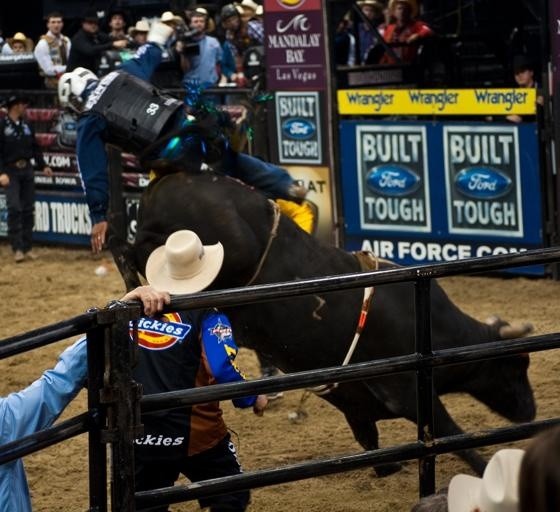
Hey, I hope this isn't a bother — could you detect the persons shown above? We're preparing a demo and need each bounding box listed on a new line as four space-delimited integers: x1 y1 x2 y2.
1 1 266 107
0 285 171 512
58 44 307 267
128 230 268 512
410 427 559 512
331 0 548 130
0 94 53 263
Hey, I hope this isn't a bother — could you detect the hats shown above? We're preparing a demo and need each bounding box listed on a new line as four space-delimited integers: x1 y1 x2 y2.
127 0 264 37
6 32 34 53
355 0 419 19
145 229 225 296
446 448 526 511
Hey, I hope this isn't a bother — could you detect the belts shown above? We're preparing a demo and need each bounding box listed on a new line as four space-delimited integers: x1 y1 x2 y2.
5 158 30 171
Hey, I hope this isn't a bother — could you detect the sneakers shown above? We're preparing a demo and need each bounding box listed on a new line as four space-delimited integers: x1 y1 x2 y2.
264 391 286 410
13 250 40 262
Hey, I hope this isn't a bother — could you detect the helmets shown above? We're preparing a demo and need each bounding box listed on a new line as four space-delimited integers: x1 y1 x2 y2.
58 67 101 122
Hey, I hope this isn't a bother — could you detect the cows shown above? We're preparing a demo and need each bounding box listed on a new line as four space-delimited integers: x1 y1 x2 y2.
107 123 536 479
